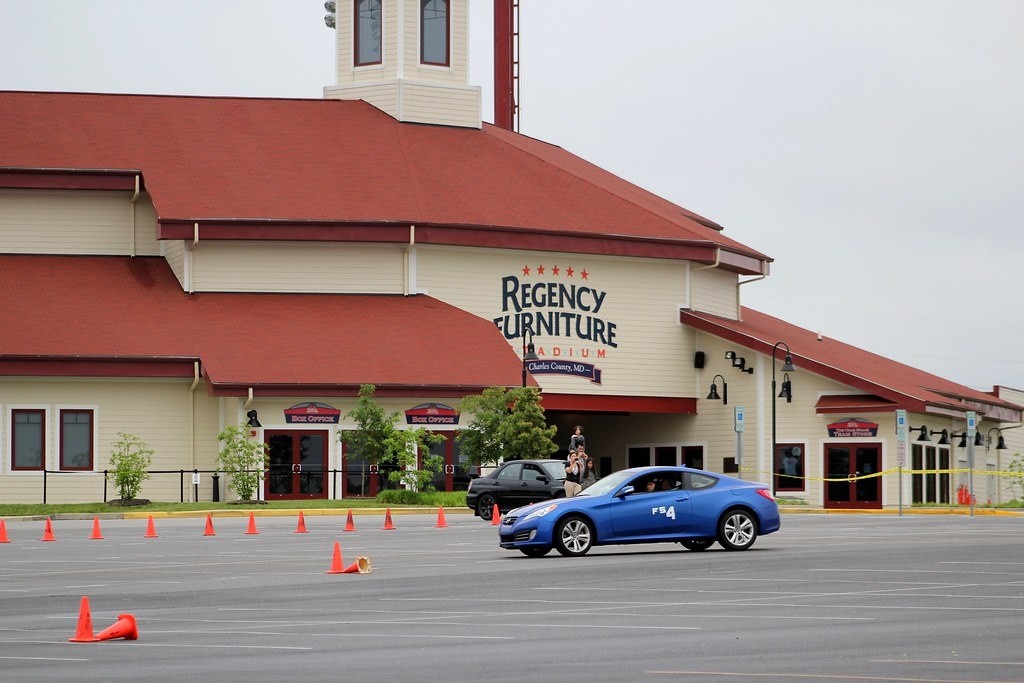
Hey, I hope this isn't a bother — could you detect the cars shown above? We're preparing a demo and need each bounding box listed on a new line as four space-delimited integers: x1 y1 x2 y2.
498 464 782 559
465 458 598 521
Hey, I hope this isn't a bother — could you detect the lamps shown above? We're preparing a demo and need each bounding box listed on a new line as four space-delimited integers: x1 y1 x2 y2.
706 375 727 405
950 432 967 447
974 431 983 446
246 410 262 428
723 351 739 368
732 358 753 375
930 429 950 444
909 425 931 442
986 428 1008 451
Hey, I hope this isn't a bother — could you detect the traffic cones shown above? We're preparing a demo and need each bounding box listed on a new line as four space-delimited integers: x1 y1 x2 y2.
326 542 345 574
244 512 261 534
489 504 500 526
434 506 449 528
40 516 57 541
380 508 396 531
293 511 310 534
344 555 373 574
143 515 159 538
88 517 106 540
0 518 11 543
94 613 139 643
68 595 96 642
201 514 216 536
342 509 358 532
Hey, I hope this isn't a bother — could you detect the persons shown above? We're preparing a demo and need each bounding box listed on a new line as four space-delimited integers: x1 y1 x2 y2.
646 477 659 492
564 425 597 497
662 477 675 490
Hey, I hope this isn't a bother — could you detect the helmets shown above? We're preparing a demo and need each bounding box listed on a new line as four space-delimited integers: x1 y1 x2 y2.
645 476 664 492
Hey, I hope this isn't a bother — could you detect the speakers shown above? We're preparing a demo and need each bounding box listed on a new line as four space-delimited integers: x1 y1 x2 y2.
695 351 704 368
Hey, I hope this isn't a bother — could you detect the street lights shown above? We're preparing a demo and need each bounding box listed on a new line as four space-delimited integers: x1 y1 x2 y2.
771 341 797 497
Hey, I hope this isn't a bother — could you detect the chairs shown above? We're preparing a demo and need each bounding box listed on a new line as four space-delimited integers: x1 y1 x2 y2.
662 479 673 490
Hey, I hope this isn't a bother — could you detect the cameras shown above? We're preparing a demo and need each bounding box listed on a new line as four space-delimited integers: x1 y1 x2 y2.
575 458 578 461
